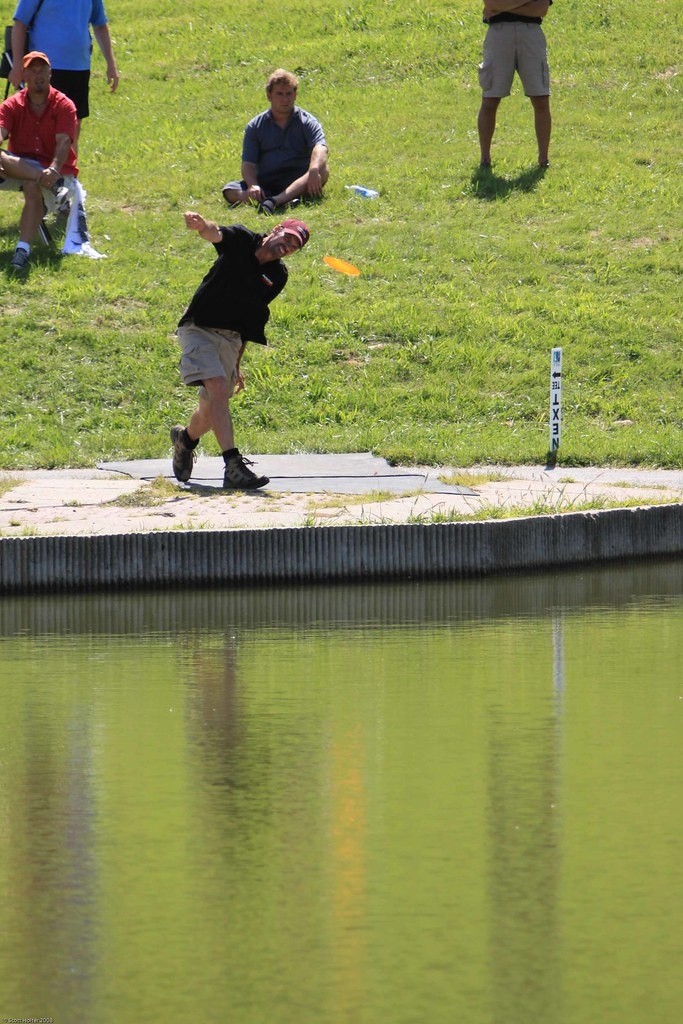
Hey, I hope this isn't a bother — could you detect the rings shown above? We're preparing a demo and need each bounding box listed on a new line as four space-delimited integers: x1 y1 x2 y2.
48 182 50 184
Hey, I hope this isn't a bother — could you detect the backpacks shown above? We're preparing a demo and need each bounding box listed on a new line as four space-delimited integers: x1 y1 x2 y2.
1 26 30 100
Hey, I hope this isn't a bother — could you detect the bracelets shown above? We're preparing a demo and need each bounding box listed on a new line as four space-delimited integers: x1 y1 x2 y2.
48 166 60 176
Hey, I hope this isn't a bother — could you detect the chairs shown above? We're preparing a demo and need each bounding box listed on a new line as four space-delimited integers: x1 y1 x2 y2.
0 25 25 102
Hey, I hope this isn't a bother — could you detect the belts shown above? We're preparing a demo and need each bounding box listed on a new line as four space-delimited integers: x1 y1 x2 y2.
489 12 542 24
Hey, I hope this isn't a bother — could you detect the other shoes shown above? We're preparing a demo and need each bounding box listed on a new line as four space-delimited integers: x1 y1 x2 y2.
286 198 300 208
258 197 275 217
10 248 28 269
50 178 69 207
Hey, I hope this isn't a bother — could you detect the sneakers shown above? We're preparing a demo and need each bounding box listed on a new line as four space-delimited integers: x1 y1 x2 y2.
170 425 197 482
223 455 269 489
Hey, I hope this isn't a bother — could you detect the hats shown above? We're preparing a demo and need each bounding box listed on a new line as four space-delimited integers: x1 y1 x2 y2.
281 219 310 251
22 51 50 68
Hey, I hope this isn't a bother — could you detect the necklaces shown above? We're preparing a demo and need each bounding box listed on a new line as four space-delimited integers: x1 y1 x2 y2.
30 101 46 107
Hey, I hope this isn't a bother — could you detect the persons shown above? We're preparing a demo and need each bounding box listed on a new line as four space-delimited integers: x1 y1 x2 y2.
170 210 310 489
7 0 120 164
0 50 80 270
222 68 329 216
477 0 553 169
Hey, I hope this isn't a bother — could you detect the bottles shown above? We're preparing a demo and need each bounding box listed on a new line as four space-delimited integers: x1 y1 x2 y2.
344 185 379 200
